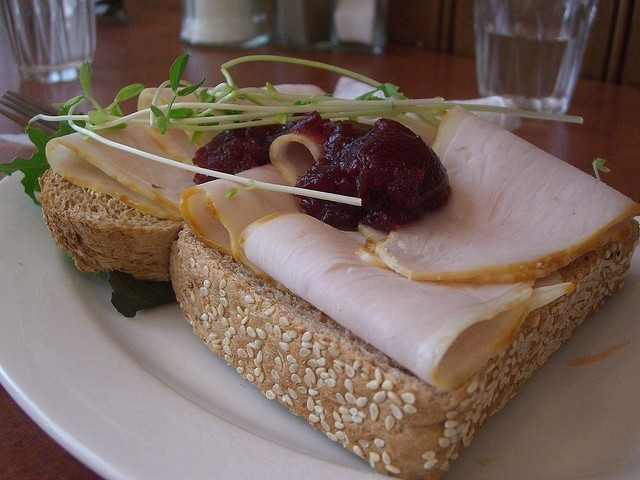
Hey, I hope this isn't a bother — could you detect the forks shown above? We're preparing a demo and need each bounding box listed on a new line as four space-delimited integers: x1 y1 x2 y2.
0 89 88 135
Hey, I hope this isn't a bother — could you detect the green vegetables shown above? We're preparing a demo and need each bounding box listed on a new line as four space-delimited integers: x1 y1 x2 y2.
1 50 584 209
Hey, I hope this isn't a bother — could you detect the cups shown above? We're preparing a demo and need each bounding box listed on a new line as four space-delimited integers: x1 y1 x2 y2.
474 2 597 114
2 1 97 83
